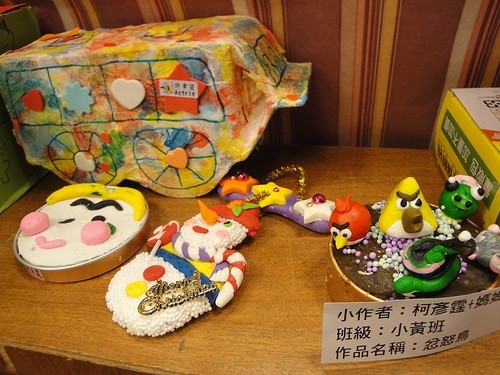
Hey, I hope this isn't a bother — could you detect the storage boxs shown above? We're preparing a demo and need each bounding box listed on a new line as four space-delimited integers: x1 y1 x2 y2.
435 87 500 231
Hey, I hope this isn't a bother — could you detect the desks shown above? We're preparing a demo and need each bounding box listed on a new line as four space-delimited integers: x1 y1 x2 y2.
0 145 500 375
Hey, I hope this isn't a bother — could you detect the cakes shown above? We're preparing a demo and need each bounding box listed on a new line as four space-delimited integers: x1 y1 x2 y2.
329 175 500 304
17 182 148 268
